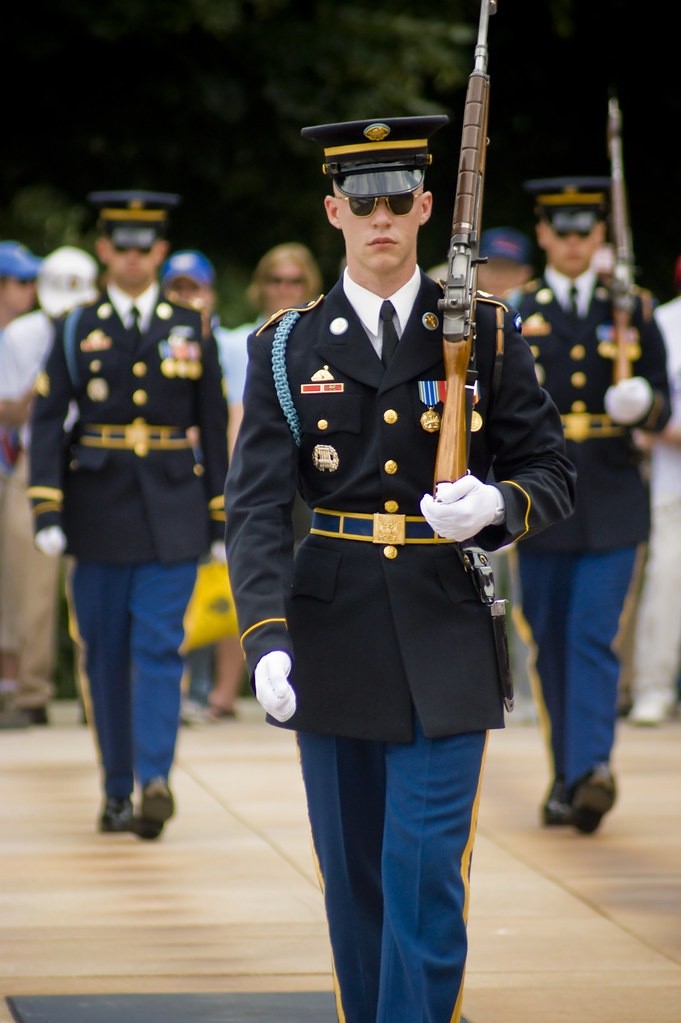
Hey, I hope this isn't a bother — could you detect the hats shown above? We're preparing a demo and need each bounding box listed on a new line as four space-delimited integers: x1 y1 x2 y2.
0 240 43 279
301 115 450 198
522 177 609 217
91 190 178 244
37 247 98 316
478 226 530 261
160 250 213 287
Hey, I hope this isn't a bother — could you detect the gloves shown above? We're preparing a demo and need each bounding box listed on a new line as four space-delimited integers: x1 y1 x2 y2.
254 650 297 723
604 376 652 424
34 524 67 557
420 474 507 542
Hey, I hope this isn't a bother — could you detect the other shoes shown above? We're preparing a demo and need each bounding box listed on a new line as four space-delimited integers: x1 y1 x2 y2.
102 798 136 831
136 780 173 838
544 777 573 822
574 772 614 833
628 689 676 725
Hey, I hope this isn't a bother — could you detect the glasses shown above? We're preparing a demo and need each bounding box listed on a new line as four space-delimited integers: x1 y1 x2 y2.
541 212 601 236
105 237 157 253
337 193 421 218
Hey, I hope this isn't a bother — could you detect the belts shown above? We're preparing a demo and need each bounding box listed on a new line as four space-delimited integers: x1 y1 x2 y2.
78 423 189 449
560 413 623 441
310 508 455 545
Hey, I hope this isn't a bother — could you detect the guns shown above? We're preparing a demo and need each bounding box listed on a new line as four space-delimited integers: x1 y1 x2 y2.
432 0 497 499
607 97 634 382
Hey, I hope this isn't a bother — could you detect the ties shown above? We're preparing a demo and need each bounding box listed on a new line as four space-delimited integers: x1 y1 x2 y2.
379 300 400 369
568 286 577 322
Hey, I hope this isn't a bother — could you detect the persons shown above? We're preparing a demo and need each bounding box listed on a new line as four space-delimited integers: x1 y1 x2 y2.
0 243 40 700
0 238 99 725
478 227 532 297
223 113 576 1023
28 190 228 839
206 241 322 718
501 176 672 835
163 251 217 320
628 294 681 726
